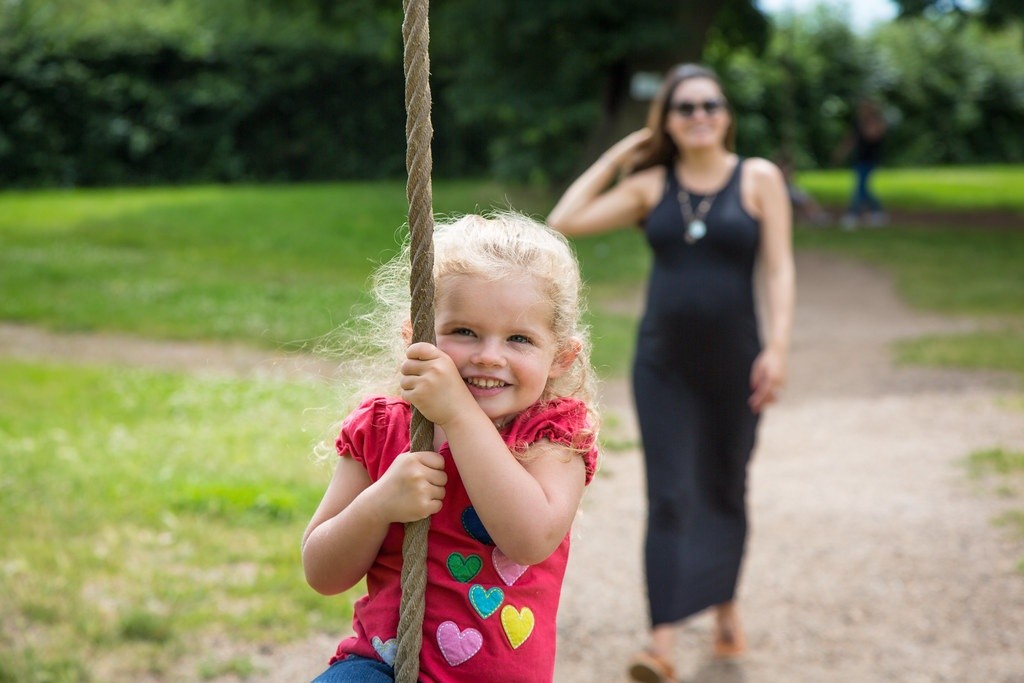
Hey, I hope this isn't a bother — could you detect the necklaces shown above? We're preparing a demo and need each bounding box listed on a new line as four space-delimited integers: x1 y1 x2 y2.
675 164 720 242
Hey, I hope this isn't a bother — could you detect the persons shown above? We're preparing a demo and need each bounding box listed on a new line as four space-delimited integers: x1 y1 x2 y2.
547 65 798 683
778 89 892 234
302 210 604 683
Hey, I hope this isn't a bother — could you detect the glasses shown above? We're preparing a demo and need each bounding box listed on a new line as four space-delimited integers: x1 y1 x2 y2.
670 100 725 117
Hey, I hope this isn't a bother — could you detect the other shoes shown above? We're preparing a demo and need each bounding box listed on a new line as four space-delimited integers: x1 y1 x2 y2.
711 619 745 658
629 655 676 683
